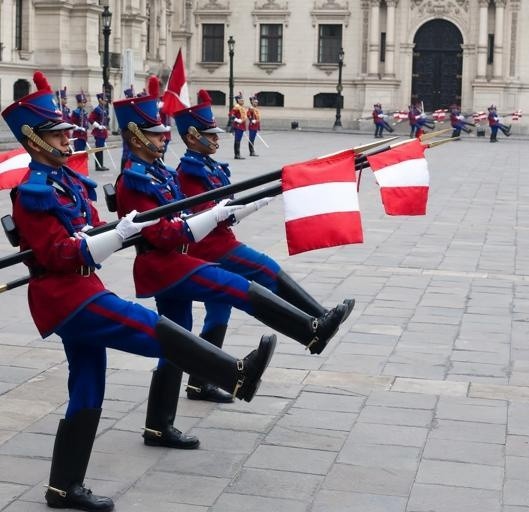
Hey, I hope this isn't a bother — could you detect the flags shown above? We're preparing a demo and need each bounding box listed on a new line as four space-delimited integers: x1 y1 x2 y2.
367 141 435 216
281 154 361 256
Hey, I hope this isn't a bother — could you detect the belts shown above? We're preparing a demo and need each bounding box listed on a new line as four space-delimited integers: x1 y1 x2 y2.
28 265 94 275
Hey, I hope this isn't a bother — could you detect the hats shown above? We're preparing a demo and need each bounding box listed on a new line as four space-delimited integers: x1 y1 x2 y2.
113 76 171 133
124 88 147 98
234 95 259 102
173 89 226 133
56 86 69 99
96 92 109 103
76 94 87 102
1 72 75 141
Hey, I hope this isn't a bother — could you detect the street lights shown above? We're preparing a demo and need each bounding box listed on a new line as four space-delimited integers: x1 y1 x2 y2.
334 46 348 135
225 35 241 132
99 6 118 138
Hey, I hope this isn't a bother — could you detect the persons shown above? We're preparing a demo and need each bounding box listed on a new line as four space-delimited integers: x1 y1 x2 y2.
89 93 110 171
174 102 355 402
122 86 171 164
371 102 512 141
247 97 261 156
71 93 89 149
114 96 349 450
55 91 71 140
1 89 277 512
230 95 246 159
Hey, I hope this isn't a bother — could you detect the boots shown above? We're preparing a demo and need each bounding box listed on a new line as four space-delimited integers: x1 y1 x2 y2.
95 147 109 171
384 124 393 132
234 141 243 159
465 122 475 126
375 128 383 138
155 314 276 402
462 126 471 132
425 124 434 129
410 130 414 138
501 125 511 136
45 408 114 511
451 130 460 141
491 133 497 142
274 270 355 336
249 140 258 156
187 323 233 402
143 370 200 449
247 282 348 354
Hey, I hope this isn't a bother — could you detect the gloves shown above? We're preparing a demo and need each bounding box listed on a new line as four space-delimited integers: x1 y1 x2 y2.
234 118 242 124
69 224 94 242
170 214 192 224
93 121 105 130
86 210 160 264
252 120 256 124
186 199 247 243
72 124 85 132
136 241 189 255
233 197 276 223
